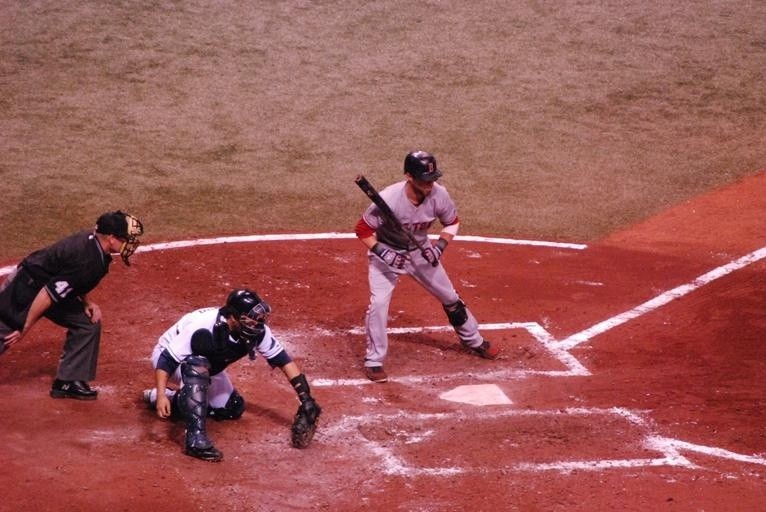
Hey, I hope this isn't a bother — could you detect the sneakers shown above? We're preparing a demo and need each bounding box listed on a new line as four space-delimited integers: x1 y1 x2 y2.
472 339 498 359
143 388 152 403
366 366 388 383
186 446 222 461
50 378 97 401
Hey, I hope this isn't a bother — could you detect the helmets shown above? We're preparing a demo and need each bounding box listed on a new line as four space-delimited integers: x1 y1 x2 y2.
226 288 271 350
404 150 443 182
95 210 144 267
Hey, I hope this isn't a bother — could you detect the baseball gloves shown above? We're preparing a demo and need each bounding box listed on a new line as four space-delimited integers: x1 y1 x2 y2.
292 398 321 449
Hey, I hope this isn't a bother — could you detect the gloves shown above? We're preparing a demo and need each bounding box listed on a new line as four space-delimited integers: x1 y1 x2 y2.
421 237 449 265
370 243 412 271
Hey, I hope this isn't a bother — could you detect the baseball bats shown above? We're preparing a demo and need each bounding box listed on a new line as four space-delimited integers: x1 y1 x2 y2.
354 175 439 267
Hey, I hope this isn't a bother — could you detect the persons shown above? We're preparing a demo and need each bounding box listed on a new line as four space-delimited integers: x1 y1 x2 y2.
0 207 145 401
143 286 323 462
352 149 501 384
